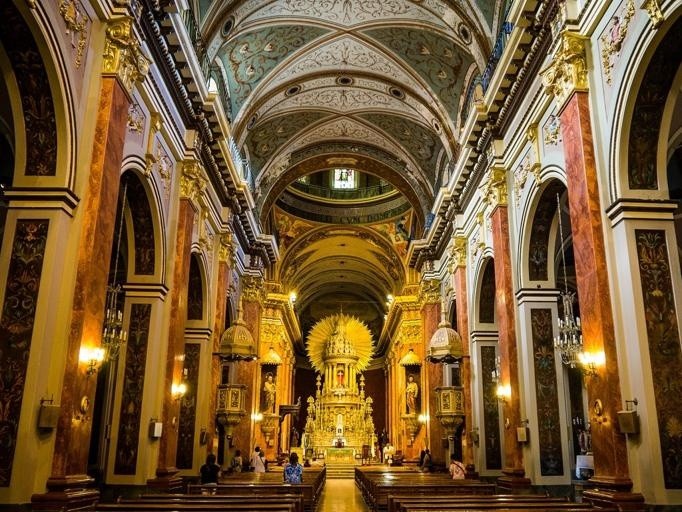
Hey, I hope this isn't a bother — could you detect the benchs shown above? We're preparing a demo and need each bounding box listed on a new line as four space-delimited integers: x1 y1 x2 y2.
91 466 326 512
355 467 601 512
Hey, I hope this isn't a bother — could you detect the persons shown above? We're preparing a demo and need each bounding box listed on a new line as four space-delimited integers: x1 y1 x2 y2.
406 375 418 414
275 447 290 466
302 458 318 467
376 431 404 466
417 450 425 467
449 453 467 480
247 446 270 473
335 439 343 448
283 453 303 484
199 454 220 496
231 450 242 473
291 426 305 447
421 449 432 473
263 376 276 414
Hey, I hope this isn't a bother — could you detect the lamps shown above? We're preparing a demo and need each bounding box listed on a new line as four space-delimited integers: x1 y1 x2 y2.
497 386 508 406
101 175 129 363
86 348 105 379
579 352 598 378
554 193 583 368
175 383 186 401
